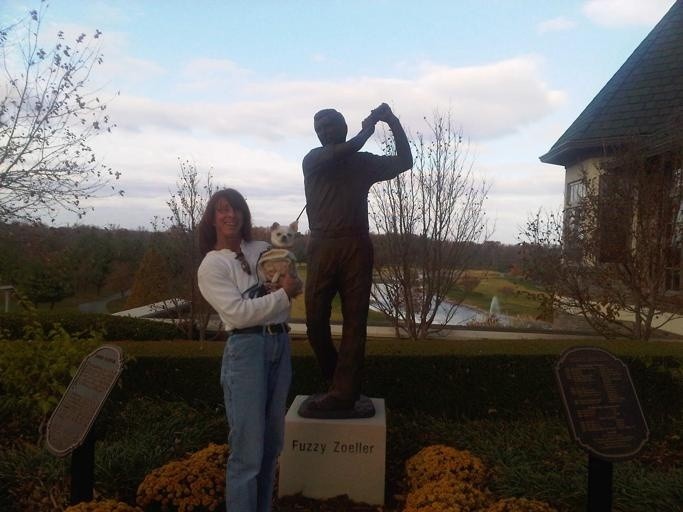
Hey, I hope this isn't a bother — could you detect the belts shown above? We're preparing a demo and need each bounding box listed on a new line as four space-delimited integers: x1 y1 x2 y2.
234 322 290 335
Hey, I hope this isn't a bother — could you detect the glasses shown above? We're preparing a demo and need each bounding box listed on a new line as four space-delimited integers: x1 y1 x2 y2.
234 251 251 275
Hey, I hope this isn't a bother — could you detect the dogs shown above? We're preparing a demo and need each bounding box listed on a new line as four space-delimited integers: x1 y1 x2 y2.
253 220 298 299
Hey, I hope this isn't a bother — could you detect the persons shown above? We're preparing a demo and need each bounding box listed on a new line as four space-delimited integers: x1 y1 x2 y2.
196 188 304 512
301 103 414 411
